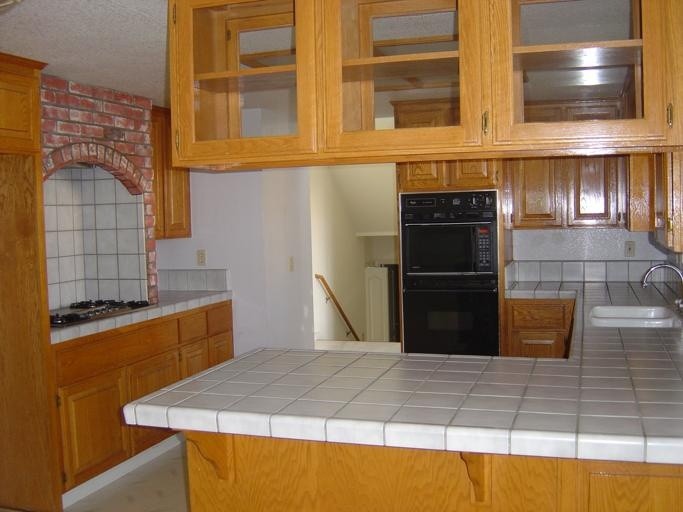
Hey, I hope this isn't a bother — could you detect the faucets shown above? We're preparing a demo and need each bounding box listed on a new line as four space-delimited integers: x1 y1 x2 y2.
640 262 683 312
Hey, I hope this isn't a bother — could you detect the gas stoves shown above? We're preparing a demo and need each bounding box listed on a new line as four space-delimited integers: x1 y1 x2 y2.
49 300 149 328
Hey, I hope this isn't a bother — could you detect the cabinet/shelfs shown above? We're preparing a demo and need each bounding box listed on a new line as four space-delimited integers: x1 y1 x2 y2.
4 53 62 507
621 65 682 252
52 300 233 493
152 105 192 240
490 1 672 159
391 96 507 228
506 298 575 359
509 98 623 228
167 2 481 172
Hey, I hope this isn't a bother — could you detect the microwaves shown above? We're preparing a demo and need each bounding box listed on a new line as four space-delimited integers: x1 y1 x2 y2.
398 191 497 273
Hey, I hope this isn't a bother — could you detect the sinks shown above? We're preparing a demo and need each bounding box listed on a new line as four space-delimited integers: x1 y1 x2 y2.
588 304 675 328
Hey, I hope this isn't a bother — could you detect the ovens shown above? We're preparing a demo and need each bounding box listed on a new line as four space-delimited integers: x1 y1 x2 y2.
399 275 500 357
